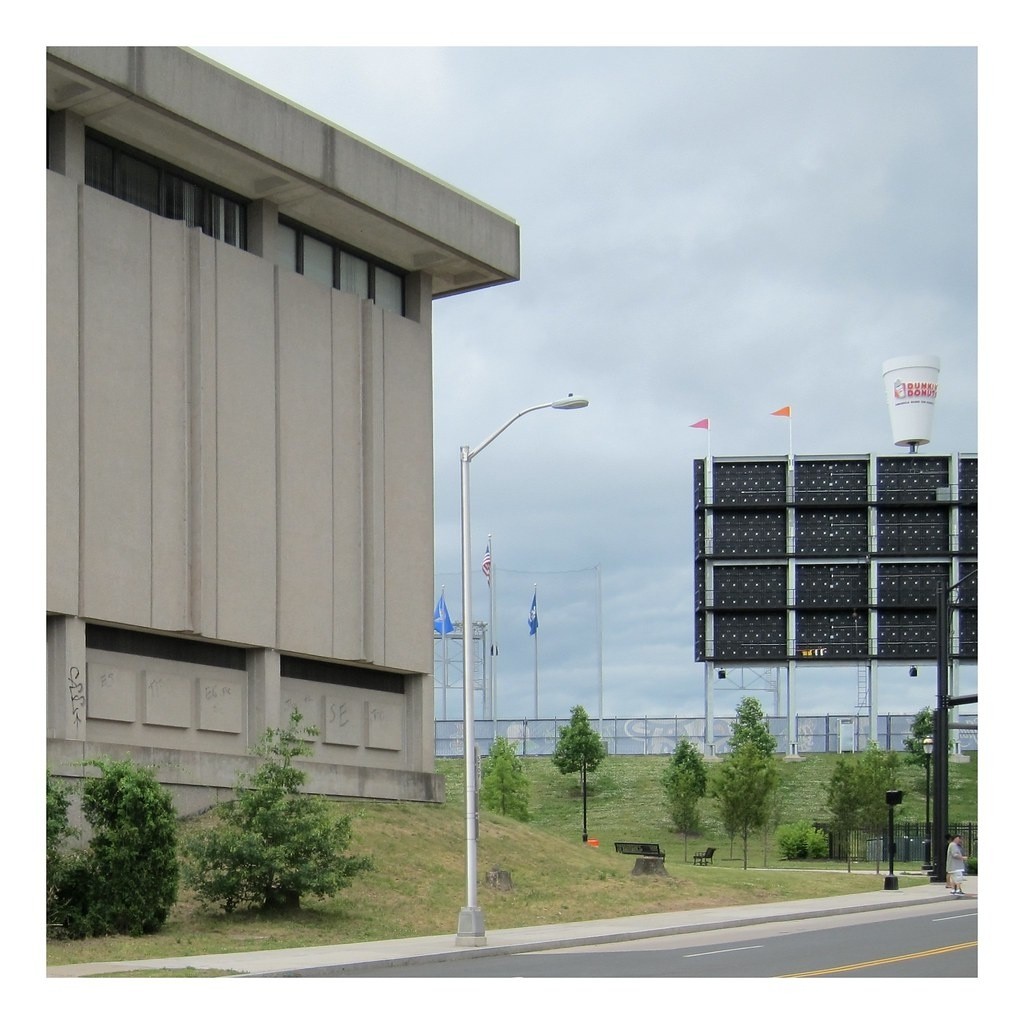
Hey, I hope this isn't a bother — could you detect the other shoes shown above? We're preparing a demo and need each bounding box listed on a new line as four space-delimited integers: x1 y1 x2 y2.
950 889 956 894
955 888 965 895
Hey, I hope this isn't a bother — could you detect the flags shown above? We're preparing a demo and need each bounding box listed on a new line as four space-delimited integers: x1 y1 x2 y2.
482 543 492 586
434 595 453 635
527 592 538 635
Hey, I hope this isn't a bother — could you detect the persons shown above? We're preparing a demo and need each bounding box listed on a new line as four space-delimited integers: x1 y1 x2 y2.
945 833 956 888
946 835 968 896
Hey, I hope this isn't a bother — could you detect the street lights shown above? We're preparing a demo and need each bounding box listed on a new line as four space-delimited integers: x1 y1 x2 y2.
455 395 592 948
922 736 933 871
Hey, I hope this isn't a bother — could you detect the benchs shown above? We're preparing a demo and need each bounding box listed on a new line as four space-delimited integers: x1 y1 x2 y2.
693 847 716 866
614 842 665 862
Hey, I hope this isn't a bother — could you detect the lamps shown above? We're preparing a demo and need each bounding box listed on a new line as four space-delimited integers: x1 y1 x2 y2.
718 667 727 679
909 665 918 677
